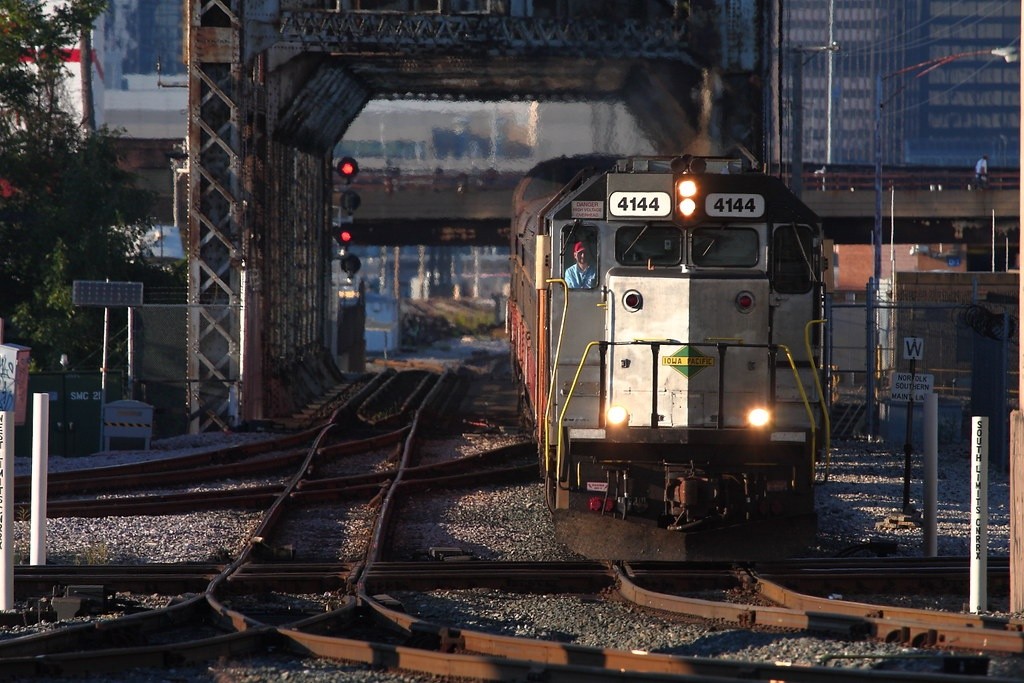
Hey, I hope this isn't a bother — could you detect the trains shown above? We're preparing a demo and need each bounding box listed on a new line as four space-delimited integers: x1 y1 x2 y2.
508 152 836 529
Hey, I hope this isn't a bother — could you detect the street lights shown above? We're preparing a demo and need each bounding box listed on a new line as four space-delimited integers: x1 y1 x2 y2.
873 48 1018 291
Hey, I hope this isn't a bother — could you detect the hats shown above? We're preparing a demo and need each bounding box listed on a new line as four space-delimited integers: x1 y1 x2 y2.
574 241 588 253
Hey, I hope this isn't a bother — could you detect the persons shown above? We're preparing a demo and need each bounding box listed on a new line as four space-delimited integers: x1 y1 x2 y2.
975 155 988 190
565 242 597 289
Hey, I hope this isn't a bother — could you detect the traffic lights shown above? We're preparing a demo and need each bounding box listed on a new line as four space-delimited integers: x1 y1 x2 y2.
341 253 362 275
341 190 360 214
338 157 358 181
336 221 359 247
